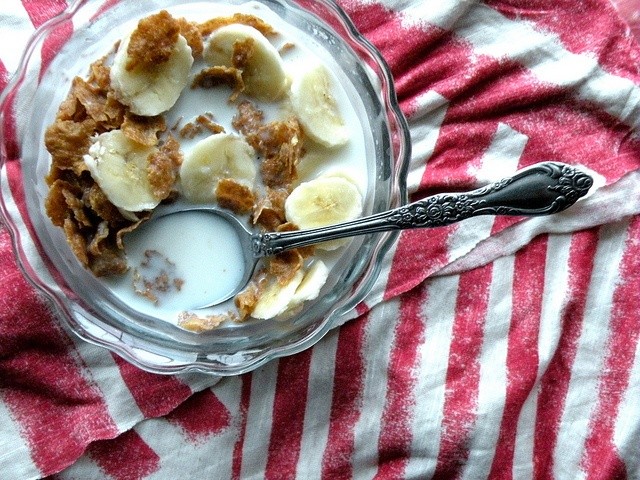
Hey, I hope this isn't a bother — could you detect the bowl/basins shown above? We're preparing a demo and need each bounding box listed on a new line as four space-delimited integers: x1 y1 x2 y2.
0 0 413 379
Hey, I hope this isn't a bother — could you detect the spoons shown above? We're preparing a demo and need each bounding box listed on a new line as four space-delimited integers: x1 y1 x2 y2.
151 163 599 309
